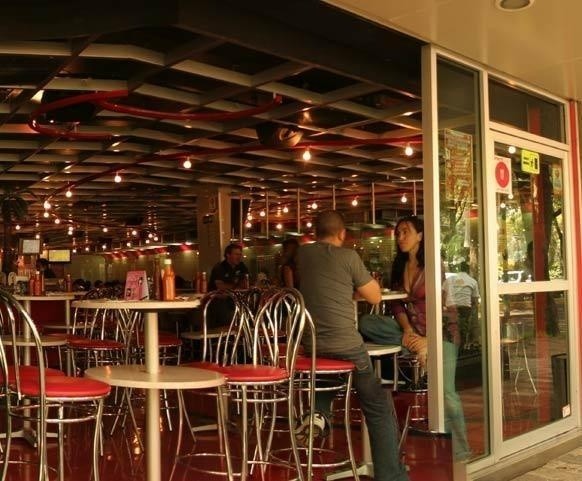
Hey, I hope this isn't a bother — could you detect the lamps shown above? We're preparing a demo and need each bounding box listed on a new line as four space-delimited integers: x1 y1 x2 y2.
302 144 312 160
245 187 408 229
404 142 413 157
41 154 191 218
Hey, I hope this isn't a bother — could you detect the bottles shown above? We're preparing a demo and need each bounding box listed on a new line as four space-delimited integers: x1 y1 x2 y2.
28 270 42 296
195 271 208 294
65 274 73 292
242 272 249 288
152 257 177 300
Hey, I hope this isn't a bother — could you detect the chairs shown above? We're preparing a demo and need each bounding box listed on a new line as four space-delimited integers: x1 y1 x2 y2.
500 316 544 393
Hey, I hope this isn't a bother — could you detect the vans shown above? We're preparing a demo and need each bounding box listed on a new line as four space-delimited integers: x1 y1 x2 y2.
498 267 534 300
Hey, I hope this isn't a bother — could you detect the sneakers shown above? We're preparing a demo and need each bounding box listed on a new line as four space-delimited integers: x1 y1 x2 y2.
294 413 325 448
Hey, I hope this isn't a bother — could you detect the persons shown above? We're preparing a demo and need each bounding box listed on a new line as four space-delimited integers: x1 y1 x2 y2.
278 239 299 319
442 260 478 351
93 279 120 290
208 244 251 289
356 215 474 467
287 209 414 481
35 259 56 279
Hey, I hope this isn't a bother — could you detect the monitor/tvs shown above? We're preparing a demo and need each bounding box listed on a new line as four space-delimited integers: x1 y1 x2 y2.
18 238 43 255
48 249 72 264
